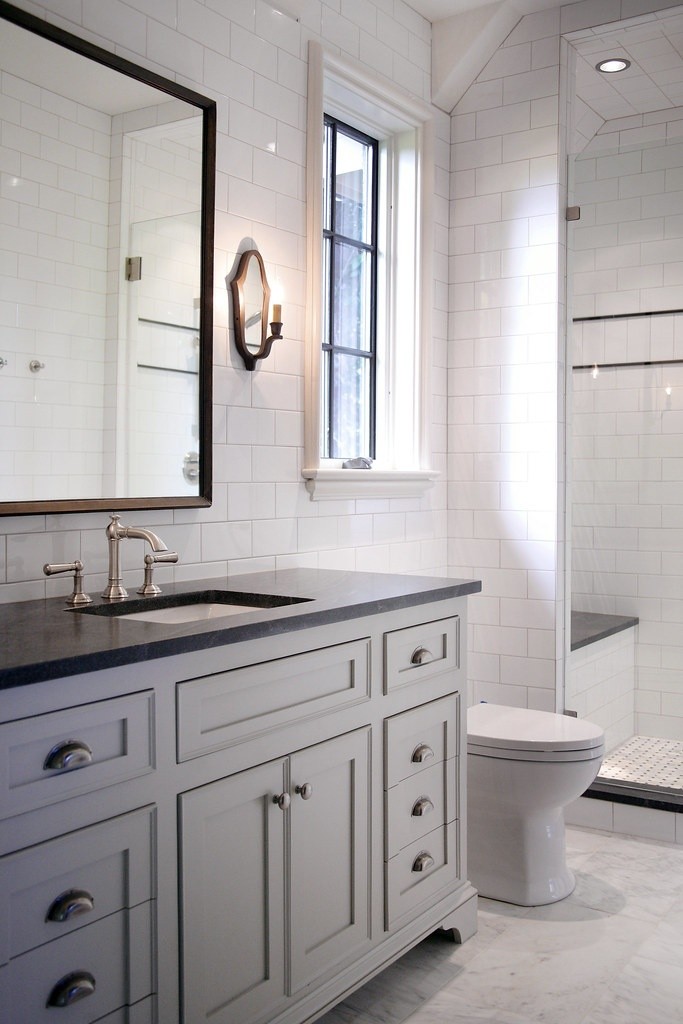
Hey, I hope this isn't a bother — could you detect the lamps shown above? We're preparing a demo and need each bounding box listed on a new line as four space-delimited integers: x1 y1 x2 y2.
269 282 284 335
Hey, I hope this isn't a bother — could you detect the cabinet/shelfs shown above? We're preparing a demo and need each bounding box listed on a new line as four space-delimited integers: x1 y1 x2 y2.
0 594 480 1024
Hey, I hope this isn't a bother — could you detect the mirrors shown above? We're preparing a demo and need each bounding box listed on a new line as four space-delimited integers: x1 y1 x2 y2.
0 0 218 517
229 249 272 371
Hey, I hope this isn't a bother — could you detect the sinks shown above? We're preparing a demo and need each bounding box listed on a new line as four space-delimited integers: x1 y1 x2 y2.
63 589 316 625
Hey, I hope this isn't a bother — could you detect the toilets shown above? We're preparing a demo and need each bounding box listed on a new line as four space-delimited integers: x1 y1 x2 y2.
466 700 606 906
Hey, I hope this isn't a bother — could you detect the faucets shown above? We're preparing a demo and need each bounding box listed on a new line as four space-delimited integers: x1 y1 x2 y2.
101 513 167 598
